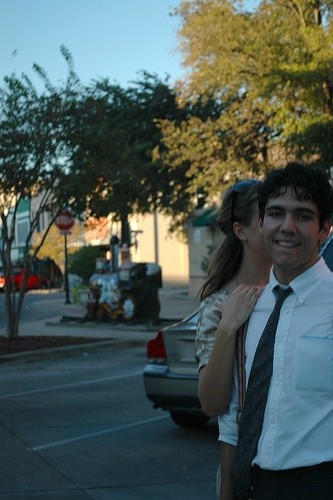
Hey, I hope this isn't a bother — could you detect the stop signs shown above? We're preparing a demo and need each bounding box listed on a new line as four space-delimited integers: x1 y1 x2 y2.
53 210 74 230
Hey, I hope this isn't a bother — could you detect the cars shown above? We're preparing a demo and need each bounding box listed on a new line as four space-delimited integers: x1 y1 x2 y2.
0 266 54 293
142 292 213 430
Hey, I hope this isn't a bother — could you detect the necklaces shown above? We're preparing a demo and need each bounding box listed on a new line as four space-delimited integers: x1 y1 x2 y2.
236 276 240 287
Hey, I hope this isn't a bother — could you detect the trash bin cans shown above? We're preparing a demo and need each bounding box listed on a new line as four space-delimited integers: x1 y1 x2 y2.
117 262 164 325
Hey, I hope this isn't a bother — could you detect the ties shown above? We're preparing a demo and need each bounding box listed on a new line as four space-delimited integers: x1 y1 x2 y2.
230 286 292 480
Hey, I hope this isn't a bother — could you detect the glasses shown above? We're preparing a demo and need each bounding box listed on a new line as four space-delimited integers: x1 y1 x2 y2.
230 180 261 224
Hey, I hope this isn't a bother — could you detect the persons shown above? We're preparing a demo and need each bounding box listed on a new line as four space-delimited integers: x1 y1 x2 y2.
113 263 160 325
216 162 333 500
120 243 131 263
192 178 272 500
